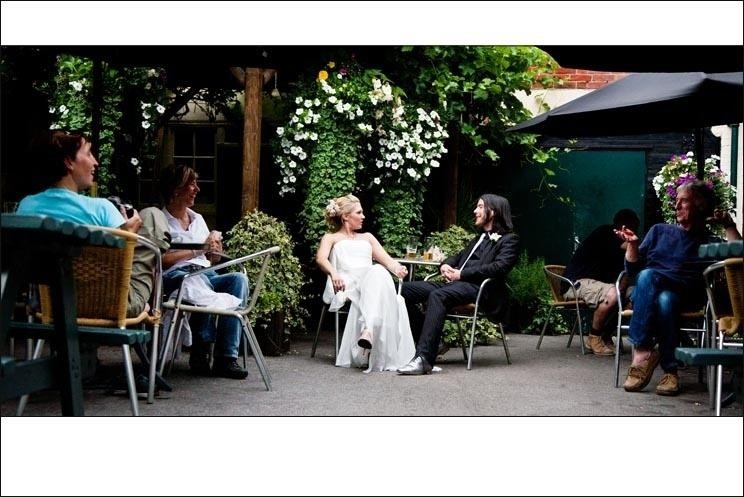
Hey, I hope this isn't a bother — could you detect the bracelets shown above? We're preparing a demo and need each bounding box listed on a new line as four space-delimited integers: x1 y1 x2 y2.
191 250 196 258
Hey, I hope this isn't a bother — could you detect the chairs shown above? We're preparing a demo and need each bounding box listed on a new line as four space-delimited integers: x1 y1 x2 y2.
1 225 743 416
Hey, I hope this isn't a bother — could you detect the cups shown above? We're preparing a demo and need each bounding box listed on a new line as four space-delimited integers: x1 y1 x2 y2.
424 244 433 260
406 244 417 259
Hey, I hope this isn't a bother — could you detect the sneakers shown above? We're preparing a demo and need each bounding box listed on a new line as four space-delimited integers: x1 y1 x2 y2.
212 355 249 381
188 342 212 379
656 373 682 397
622 349 659 392
584 335 619 356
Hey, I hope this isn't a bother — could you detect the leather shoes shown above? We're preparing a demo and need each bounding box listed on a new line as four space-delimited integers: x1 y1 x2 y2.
395 355 432 375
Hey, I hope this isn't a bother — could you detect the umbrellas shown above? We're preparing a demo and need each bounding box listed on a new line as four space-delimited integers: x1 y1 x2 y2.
504 71 743 182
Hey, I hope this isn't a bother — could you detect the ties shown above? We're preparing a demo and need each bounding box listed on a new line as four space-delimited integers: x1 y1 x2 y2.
458 233 486 271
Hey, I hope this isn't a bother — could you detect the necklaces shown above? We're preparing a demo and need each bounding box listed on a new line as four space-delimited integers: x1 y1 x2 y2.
167 205 190 231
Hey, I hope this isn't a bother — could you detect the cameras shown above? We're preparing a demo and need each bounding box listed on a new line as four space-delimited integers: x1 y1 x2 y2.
108 195 134 219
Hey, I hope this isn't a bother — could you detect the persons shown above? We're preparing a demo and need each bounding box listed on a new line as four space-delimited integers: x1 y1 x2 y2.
159 164 249 379
560 208 642 356
314 195 441 375
14 131 160 400
613 181 742 396
394 193 520 376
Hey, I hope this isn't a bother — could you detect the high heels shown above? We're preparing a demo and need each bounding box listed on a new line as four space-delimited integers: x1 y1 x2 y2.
358 329 373 357
